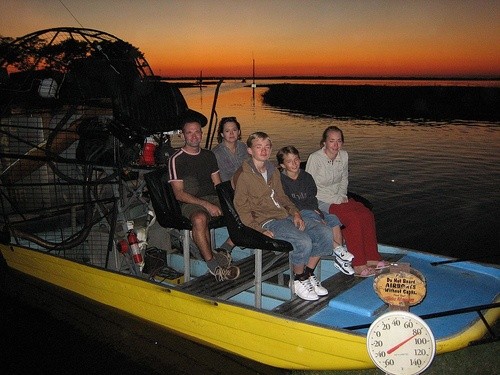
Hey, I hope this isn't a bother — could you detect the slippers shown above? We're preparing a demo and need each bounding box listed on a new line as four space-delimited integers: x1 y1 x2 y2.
353 266 377 277
371 259 391 270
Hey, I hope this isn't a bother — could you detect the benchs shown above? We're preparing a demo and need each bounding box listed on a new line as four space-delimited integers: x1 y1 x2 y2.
143 167 227 283
215 160 374 309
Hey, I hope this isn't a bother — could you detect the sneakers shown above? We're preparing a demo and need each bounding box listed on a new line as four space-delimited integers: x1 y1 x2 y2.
333 256 355 275
305 269 329 296
207 266 240 281
334 246 355 263
288 274 321 301
210 247 233 266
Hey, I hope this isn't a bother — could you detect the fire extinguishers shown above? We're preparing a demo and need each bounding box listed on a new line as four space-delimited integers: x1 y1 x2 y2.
126 229 142 264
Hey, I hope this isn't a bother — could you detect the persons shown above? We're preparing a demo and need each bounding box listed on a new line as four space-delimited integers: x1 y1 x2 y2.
276 145 355 276
212 117 251 182
305 126 391 278
167 117 241 281
231 132 334 301
